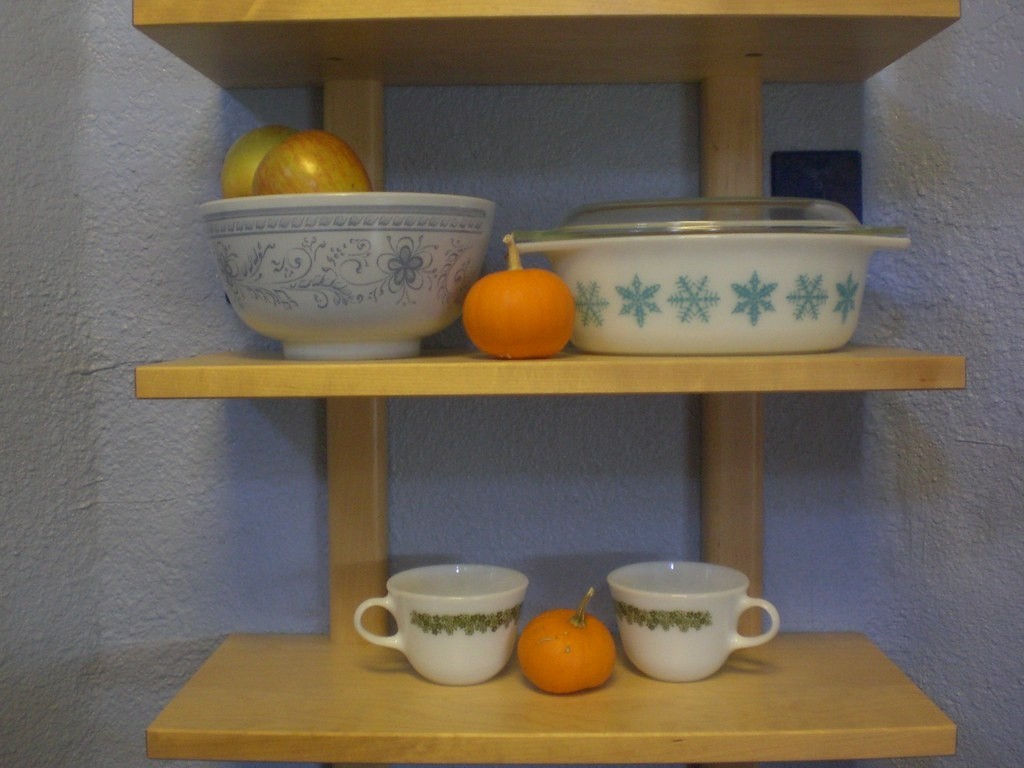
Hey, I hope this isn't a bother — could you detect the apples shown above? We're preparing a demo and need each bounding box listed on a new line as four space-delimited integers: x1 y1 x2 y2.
218 122 372 198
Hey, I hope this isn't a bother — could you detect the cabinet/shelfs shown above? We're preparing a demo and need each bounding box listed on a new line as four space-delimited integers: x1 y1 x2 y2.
132 0 964 768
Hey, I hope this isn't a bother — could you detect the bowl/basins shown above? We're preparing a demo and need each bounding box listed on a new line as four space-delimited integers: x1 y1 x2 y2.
200 192 496 360
514 233 912 355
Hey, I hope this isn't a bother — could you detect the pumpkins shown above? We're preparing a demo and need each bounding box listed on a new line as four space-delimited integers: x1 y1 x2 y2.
461 233 575 360
517 587 616 693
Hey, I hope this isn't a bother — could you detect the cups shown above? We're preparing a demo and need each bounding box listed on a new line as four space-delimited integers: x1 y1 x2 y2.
606 560 781 683
353 563 530 686
